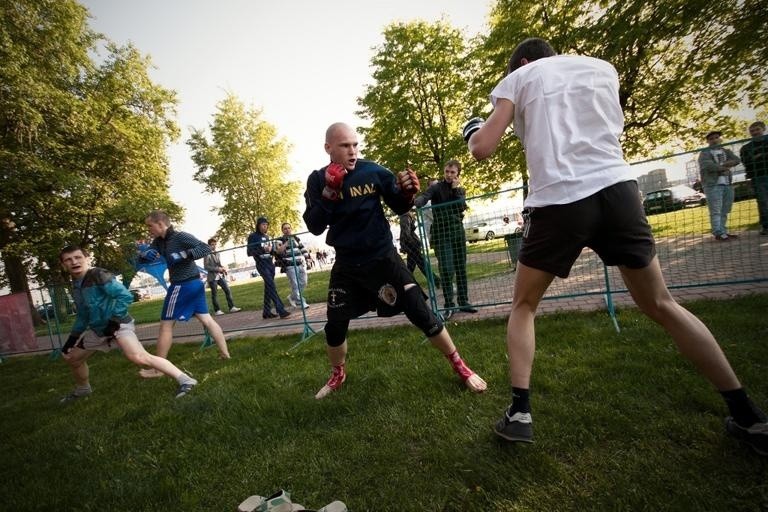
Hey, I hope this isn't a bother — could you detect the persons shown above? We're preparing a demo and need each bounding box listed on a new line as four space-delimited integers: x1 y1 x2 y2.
308 247 336 269
399 210 441 290
35 298 48 311
461 37 768 445
693 178 704 193
246 217 291 319
302 122 488 399
420 177 458 295
139 210 231 379
275 223 311 310
59 245 199 403
414 159 478 322
696 130 741 243
203 239 242 316
738 120 768 235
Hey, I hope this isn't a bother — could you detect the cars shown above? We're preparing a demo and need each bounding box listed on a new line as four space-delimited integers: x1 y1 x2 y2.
643 185 706 215
36 300 78 320
130 289 149 299
731 173 756 202
464 218 524 241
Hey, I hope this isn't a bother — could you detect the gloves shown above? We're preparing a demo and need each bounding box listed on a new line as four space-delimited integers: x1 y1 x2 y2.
397 168 421 205
168 250 188 265
461 116 486 141
141 247 159 264
323 165 346 199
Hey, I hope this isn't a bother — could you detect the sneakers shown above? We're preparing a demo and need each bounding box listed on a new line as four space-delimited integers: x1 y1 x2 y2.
214 310 225 316
287 294 298 308
296 304 309 310
719 411 768 454
487 415 537 444
229 306 243 312
716 235 732 242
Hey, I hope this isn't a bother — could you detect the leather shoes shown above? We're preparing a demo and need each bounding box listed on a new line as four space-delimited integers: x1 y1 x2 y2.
460 303 478 312
443 306 457 320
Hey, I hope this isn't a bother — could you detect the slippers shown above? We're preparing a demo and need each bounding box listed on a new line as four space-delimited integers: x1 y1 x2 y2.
175 381 198 402
